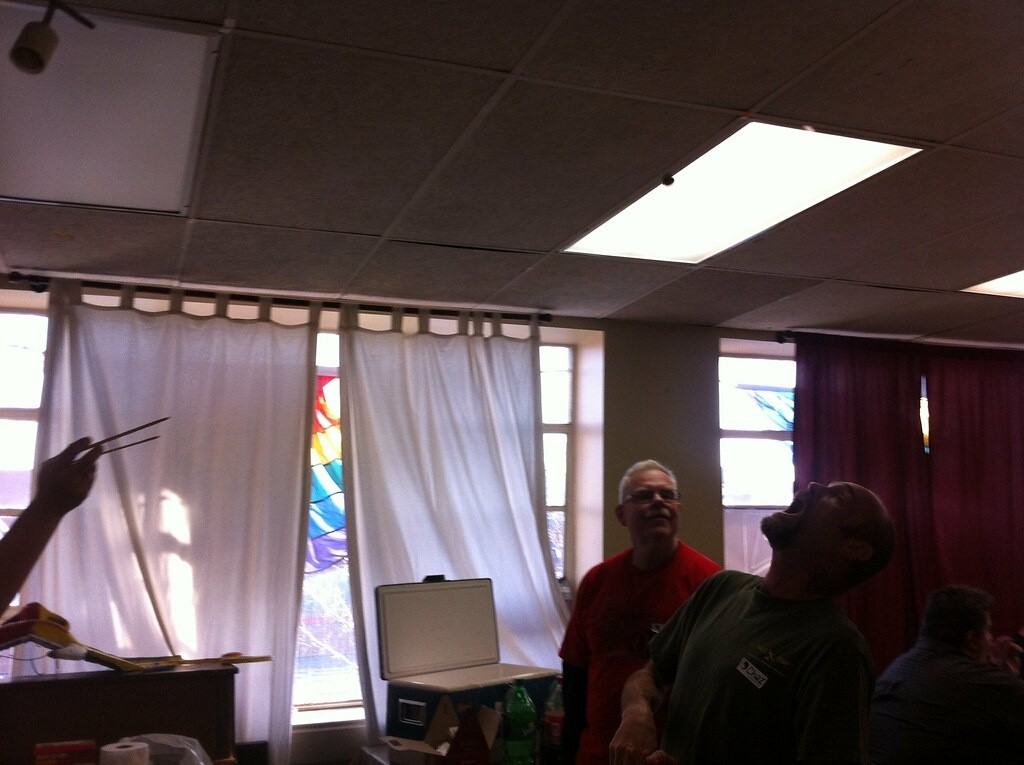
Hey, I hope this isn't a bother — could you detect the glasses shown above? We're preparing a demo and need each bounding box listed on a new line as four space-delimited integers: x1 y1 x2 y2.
622 489 684 504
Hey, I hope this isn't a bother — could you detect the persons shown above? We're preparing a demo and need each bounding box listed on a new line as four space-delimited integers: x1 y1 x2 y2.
606 479 895 765
558 458 724 765
864 581 1024 765
0 436 105 621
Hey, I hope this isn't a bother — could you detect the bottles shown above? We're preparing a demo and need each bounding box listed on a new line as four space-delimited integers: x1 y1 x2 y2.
558 575 577 614
504 679 539 765
544 676 568 765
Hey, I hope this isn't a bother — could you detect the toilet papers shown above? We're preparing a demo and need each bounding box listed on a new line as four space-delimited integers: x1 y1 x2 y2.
99 743 151 765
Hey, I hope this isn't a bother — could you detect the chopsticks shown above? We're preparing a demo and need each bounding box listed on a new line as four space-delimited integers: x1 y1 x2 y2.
74 416 172 464
